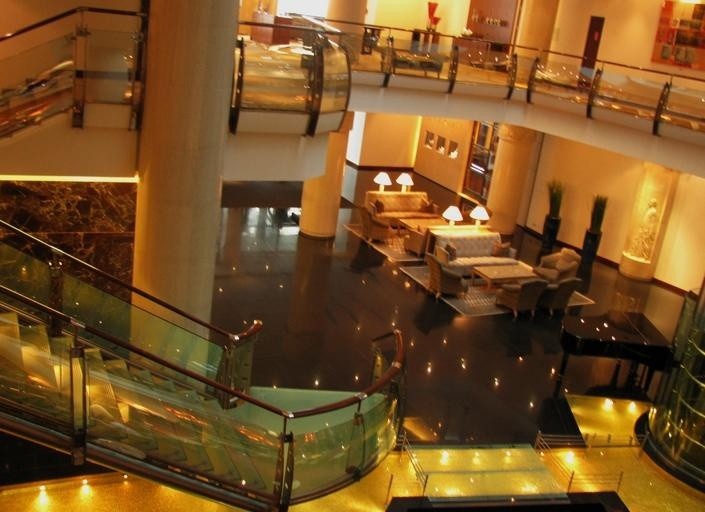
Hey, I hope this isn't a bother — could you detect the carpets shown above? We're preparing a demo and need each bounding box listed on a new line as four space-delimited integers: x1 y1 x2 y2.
220 179 356 210
342 222 426 263
398 261 596 318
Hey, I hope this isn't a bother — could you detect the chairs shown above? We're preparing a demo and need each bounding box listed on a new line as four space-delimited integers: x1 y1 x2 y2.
358 187 585 319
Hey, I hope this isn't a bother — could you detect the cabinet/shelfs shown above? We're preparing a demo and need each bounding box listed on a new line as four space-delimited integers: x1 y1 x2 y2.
452 32 514 70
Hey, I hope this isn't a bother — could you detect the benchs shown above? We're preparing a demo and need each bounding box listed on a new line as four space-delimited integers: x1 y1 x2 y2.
380 46 449 80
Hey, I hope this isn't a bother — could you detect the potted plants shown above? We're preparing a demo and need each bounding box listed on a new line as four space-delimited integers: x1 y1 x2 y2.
583 195 608 264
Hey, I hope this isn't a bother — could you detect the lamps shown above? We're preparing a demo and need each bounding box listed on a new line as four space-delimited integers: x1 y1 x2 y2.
396 172 414 192
373 171 393 191
